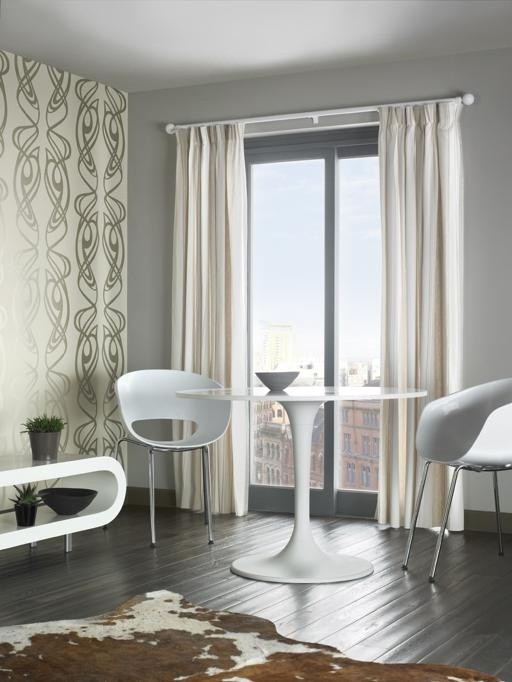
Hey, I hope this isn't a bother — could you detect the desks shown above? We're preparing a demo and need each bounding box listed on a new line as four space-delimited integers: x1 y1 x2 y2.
176 385 428 585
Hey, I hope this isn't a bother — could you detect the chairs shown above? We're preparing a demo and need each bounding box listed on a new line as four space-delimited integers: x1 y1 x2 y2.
400 377 512 583
114 369 232 548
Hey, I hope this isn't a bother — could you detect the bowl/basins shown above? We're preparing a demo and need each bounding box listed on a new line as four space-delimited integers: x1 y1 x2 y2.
38 487 98 516
256 372 299 394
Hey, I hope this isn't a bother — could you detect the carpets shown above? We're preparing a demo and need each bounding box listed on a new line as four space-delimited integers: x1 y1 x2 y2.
0 589 504 682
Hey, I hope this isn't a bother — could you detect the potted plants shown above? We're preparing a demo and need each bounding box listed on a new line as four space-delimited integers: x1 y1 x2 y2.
20 416 64 460
8 482 46 526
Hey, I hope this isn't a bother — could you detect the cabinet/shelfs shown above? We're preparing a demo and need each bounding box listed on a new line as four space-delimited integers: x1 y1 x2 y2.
1 454 127 553
